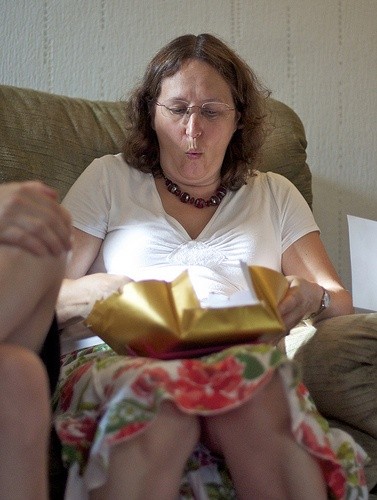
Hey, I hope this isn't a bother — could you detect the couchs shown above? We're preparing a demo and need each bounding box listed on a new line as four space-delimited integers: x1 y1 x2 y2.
0 84 377 500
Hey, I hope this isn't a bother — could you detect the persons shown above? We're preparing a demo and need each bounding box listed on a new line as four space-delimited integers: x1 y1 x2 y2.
0 180 73 499
60 33 372 498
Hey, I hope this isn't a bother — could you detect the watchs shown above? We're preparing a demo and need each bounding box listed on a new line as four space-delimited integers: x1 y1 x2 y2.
310 286 330 317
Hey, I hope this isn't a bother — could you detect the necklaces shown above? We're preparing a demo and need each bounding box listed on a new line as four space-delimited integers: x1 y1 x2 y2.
162 175 226 208
